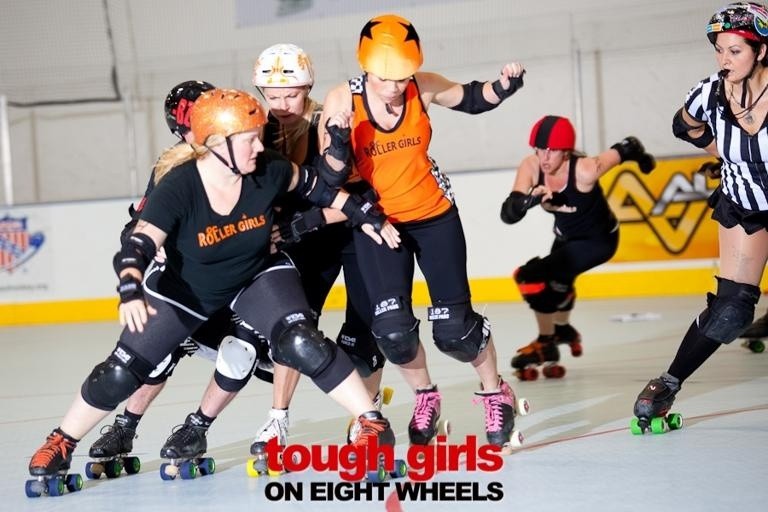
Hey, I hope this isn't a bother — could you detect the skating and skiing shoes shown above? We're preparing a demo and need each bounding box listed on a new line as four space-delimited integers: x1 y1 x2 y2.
510 333 565 380
158 413 217 482
344 411 406 487
245 407 299 480
548 322 583 360
629 369 683 436
86 413 140 482
405 384 451 447
24 427 84 499
347 384 395 438
470 375 530 455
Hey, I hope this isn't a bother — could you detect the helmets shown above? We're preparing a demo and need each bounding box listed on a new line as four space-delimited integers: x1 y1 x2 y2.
530 115 578 152
184 88 268 176
250 41 317 125
162 79 214 141
706 0 766 45
356 13 425 116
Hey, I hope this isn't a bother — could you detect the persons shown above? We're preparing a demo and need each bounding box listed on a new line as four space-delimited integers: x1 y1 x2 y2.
23 86 402 500
314 11 531 455
244 41 385 482
85 79 268 484
630 0 768 434
499 114 656 382
